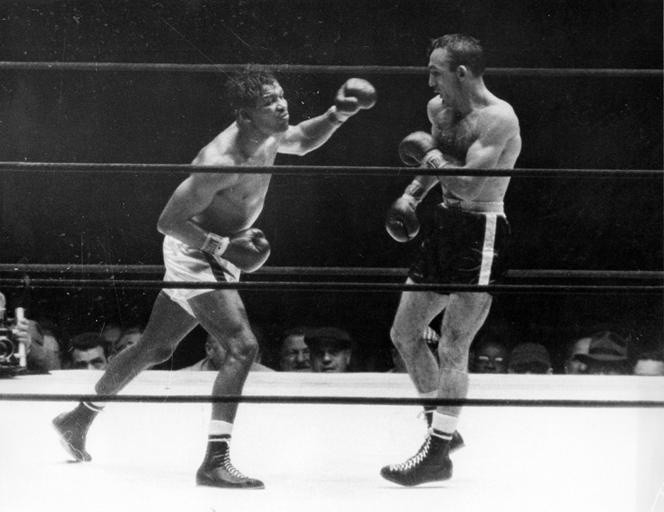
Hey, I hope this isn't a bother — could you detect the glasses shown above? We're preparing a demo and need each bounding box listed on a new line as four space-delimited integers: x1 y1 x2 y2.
511 362 549 374
477 354 505 364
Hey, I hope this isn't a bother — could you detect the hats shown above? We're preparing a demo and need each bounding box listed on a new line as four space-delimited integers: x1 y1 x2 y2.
571 331 627 362
422 325 440 343
304 326 351 350
509 343 550 365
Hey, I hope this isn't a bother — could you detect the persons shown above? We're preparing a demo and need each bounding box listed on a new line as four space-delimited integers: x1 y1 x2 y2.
380 32 526 489
48 62 381 491
0 311 662 377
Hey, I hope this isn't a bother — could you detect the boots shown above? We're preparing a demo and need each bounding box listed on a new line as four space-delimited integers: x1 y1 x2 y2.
195 434 265 490
379 405 466 488
50 401 104 462
